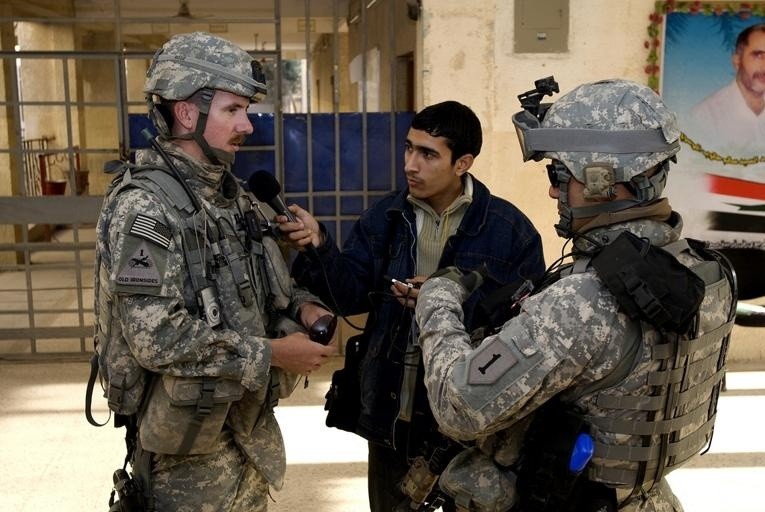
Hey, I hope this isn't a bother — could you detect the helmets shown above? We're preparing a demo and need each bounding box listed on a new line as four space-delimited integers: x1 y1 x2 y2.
143 31 267 102
541 79 680 200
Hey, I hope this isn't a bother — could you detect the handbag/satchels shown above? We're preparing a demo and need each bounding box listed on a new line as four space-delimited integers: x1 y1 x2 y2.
323 333 366 434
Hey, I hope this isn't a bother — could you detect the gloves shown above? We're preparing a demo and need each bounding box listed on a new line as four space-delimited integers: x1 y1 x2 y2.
425 265 484 302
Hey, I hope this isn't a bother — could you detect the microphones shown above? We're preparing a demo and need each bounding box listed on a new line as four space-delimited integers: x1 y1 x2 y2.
245 168 319 258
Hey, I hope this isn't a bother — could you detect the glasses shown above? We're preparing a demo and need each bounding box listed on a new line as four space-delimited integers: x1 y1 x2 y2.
310 314 337 345
545 163 570 186
512 103 666 163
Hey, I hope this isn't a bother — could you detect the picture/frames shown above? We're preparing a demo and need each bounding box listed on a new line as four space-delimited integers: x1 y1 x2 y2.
651 3 765 250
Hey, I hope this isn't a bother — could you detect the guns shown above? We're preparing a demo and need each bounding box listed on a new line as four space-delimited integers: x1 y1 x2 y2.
113 470 142 511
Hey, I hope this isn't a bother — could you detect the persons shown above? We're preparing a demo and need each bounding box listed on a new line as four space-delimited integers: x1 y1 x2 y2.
412 75 741 511
267 100 547 509
89 29 339 511
689 25 765 179
